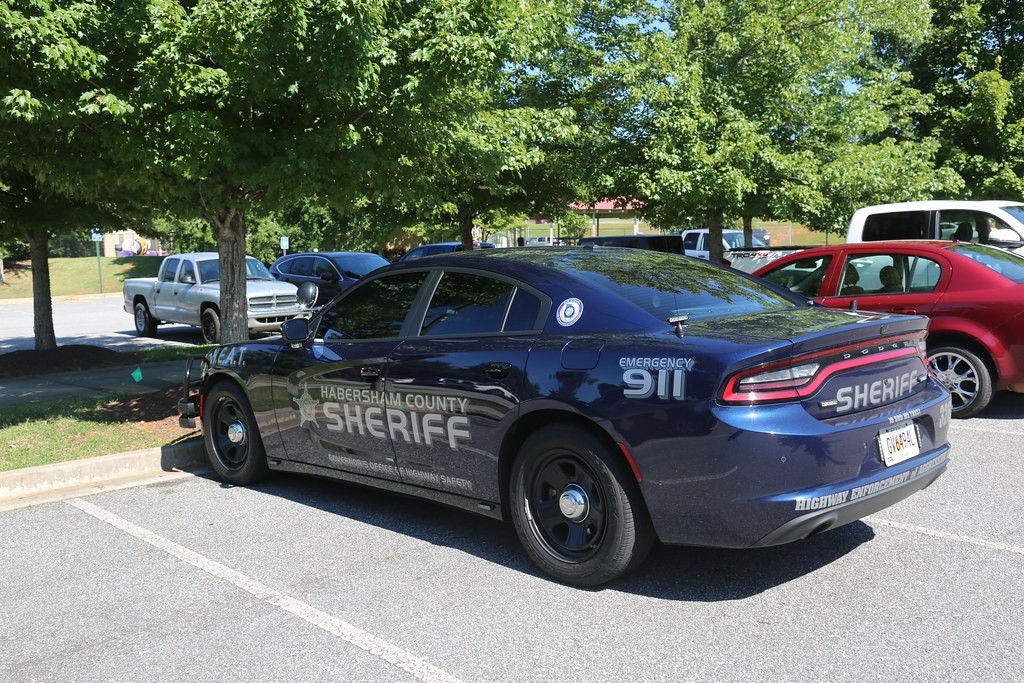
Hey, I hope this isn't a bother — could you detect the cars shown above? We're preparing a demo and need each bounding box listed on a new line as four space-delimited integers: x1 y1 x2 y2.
921 221 979 241
749 239 1024 419
750 227 770 245
267 251 393 309
177 244 952 592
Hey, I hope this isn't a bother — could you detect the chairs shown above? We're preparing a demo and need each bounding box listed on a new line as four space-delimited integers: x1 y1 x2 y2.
954 222 973 242
878 265 903 293
205 270 219 280
840 263 864 296
424 295 474 334
473 292 528 330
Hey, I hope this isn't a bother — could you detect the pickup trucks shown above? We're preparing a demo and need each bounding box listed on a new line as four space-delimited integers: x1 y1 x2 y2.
123 250 314 345
726 200 1024 292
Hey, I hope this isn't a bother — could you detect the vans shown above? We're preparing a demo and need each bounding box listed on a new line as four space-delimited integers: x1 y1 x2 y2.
682 228 770 264
397 242 495 263
525 235 558 247
